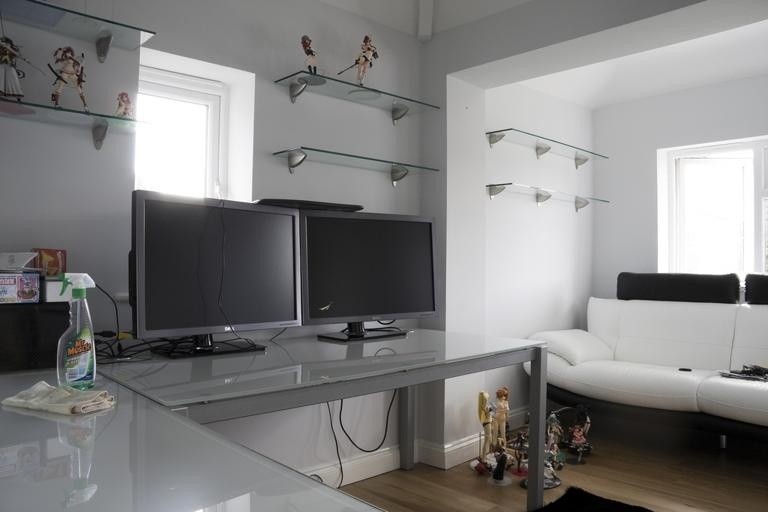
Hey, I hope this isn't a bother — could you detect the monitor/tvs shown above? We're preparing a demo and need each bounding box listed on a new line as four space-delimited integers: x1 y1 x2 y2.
303 210 438 343
128 190 301 359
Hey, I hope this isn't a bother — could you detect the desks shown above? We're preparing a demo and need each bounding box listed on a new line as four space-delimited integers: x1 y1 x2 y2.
3 365 386 512
93 329 547 509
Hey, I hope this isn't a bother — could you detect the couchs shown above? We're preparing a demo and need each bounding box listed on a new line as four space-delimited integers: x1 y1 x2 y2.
522 272 768 446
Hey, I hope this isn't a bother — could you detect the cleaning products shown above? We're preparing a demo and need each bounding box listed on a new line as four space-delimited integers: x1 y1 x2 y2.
56 272 96 390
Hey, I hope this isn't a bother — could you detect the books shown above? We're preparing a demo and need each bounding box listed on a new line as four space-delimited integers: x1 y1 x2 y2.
0 251 42 274
721 372 768 382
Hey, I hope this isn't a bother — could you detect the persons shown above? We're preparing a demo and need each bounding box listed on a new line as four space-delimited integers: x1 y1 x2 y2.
114 92 131 118
0 36 44 103
299 34 317 75
47 44 88 113
470 385 593 489
337 35 380 87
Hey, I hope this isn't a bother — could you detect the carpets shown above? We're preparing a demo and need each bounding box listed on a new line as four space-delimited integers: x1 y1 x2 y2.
544 486 654 511
